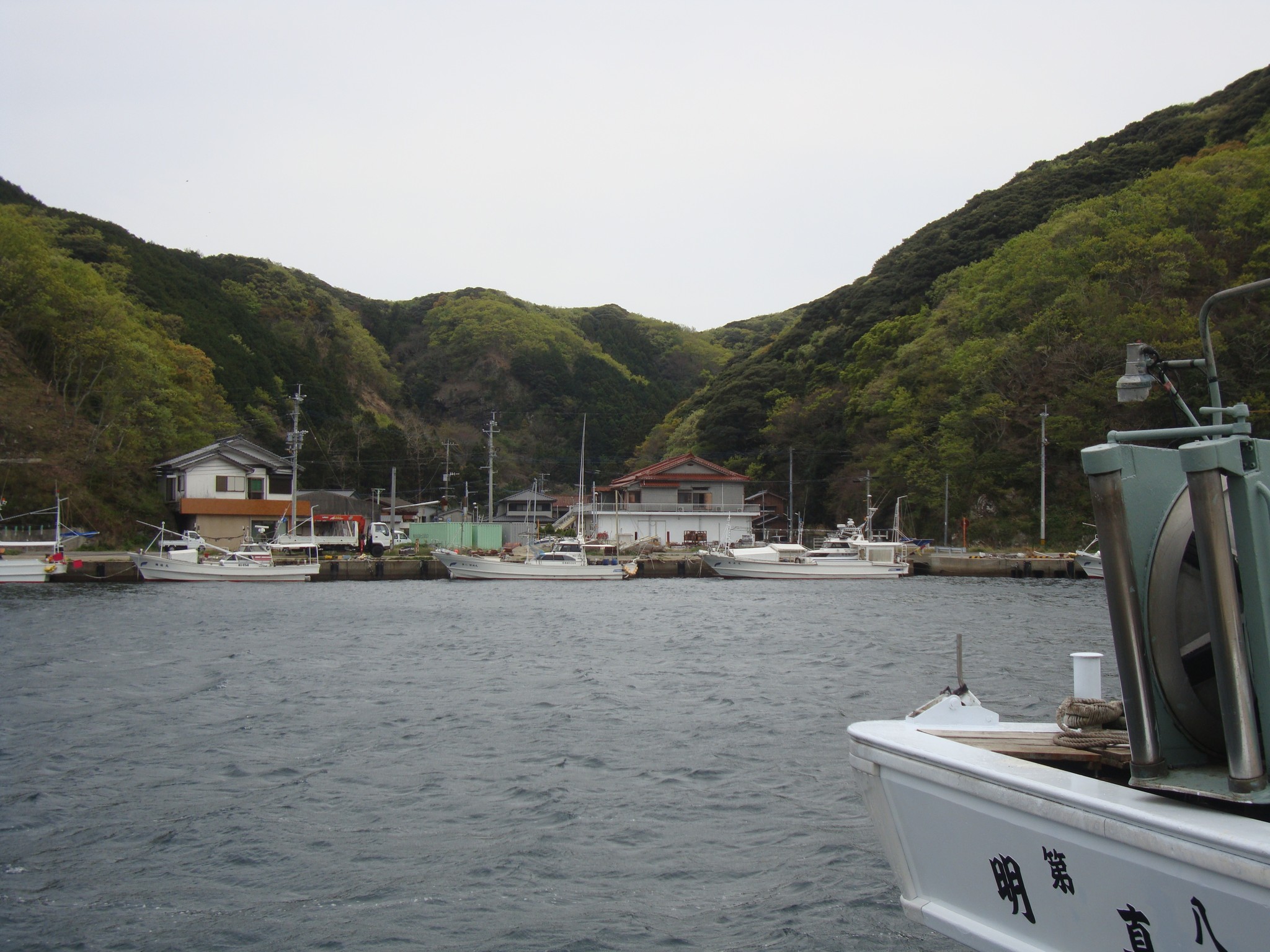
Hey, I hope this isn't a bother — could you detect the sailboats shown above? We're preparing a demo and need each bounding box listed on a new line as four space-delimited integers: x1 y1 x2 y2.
0 457 101 584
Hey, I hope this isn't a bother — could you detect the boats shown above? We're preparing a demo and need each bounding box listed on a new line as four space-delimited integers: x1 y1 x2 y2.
695 494 935 581
430 533 638 581
851 274 1269 952
1067 520 1105 580
127 514 321 584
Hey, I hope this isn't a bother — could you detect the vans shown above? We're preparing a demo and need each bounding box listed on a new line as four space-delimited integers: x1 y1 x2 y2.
376 530 412 547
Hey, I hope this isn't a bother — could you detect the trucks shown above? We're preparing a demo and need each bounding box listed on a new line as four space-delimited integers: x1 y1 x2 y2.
277 513 393 558
